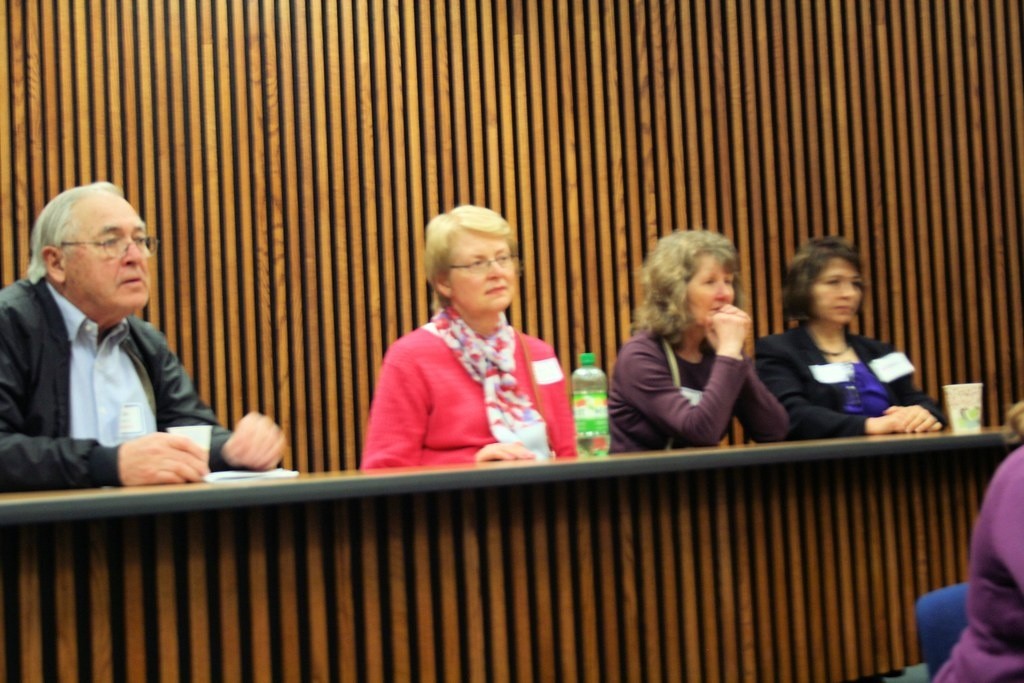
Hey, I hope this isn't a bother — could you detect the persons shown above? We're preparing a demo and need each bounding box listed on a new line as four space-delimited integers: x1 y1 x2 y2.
753 236 947 439
607 230 789 456
0 182 284 491
931 401 1024 683
361 205 578 470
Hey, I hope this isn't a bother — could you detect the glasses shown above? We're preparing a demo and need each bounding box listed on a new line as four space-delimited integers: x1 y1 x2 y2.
447 254 520 276
60 236 157 258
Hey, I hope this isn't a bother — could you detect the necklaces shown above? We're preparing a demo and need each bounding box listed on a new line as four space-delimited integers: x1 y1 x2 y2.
821 347 850 356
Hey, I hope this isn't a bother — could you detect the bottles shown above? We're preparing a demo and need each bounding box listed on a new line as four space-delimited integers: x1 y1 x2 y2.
570 352 611 458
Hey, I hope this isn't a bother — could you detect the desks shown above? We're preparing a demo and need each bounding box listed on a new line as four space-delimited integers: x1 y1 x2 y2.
0 429 1013 683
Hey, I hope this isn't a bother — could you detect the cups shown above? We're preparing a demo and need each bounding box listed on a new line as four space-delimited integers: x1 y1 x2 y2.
942 382 984 436
168 425 212 478
